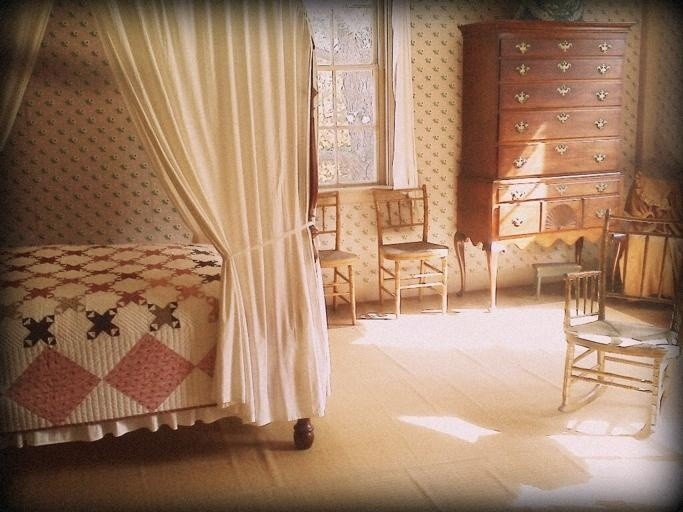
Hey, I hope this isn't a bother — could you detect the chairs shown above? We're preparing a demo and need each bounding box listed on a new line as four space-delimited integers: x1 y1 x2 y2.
558 213 682 432
308 186 450 327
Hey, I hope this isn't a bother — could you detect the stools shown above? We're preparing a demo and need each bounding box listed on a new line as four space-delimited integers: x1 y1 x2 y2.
530 262 582 299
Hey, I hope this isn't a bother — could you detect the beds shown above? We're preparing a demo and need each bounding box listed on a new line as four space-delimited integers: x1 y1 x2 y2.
1 242 324 448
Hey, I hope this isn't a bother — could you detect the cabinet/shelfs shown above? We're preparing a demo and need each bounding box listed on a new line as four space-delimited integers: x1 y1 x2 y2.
452 20 625 315
